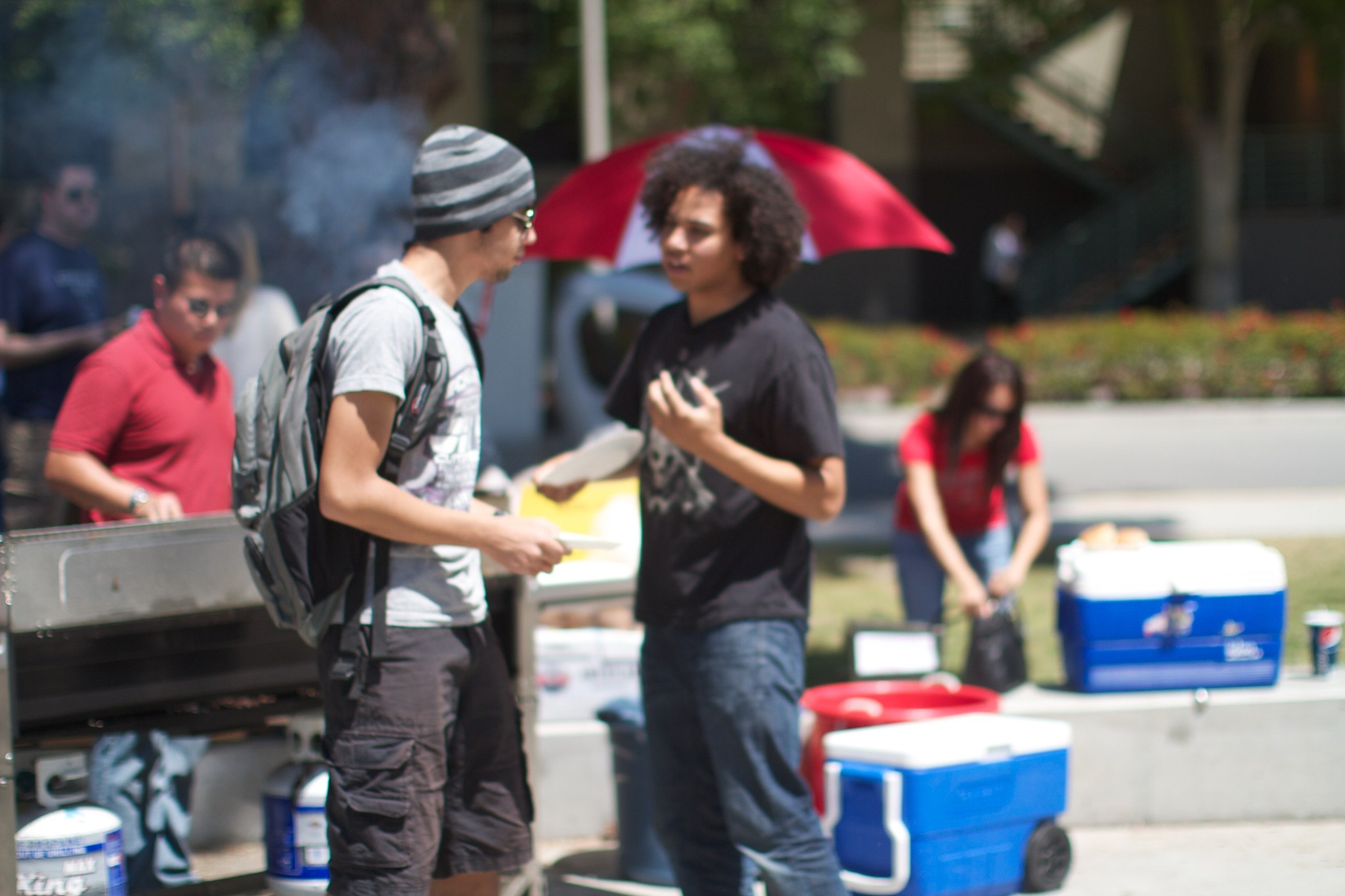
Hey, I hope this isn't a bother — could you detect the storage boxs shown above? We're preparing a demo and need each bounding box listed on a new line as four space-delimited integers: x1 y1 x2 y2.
1056 539 1288 692
819 712 1071 896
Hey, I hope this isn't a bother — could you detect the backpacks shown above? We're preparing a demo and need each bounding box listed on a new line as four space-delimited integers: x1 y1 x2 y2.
231 277 447 660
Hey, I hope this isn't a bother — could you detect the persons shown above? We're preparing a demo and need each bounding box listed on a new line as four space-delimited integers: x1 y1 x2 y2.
889 350 1054 692
232 121 578 896
0 155 307 896
531 121 859 896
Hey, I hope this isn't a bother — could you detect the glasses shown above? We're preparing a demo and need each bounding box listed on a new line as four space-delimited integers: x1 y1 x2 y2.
512 209 535 234
68 185 103 203
175 289 234 317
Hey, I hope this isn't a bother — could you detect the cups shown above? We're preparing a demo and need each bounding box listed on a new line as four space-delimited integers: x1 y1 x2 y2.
1303 610 1343 674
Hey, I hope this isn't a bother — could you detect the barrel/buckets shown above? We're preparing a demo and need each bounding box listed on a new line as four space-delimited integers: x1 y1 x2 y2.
594 696 679 887
799 673 1003 815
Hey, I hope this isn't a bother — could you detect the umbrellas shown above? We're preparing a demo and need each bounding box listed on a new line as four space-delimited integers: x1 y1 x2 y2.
526 121 955 277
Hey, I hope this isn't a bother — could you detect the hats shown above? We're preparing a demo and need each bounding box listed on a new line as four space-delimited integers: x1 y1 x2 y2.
411 122 536 240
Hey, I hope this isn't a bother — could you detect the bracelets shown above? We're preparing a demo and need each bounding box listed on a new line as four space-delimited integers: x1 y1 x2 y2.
125 486 152 516
